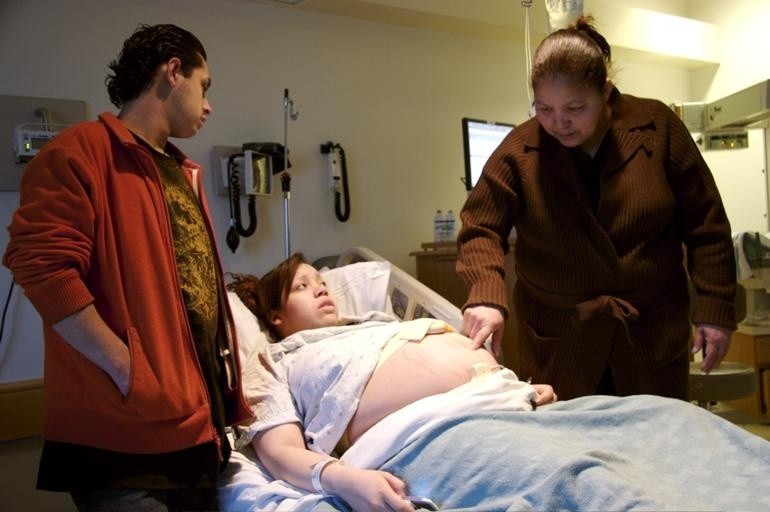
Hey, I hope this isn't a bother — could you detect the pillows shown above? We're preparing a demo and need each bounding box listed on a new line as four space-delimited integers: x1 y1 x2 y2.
224 260 391 375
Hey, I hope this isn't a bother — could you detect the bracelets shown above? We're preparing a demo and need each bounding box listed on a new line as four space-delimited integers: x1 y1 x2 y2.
310 455 345 498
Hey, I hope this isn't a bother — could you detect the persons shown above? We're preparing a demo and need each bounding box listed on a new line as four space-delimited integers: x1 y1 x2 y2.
3 21 257 512
454 13 736 400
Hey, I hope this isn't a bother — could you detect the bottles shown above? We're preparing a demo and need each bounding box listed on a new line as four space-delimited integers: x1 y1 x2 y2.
446 210 456 240
434 209 445 241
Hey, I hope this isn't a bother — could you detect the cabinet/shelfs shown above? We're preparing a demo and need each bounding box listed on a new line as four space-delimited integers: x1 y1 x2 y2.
409 240 521 381
714 320 770 421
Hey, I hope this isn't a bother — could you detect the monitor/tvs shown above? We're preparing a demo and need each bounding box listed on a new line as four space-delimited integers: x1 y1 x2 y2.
461 116 517 192
